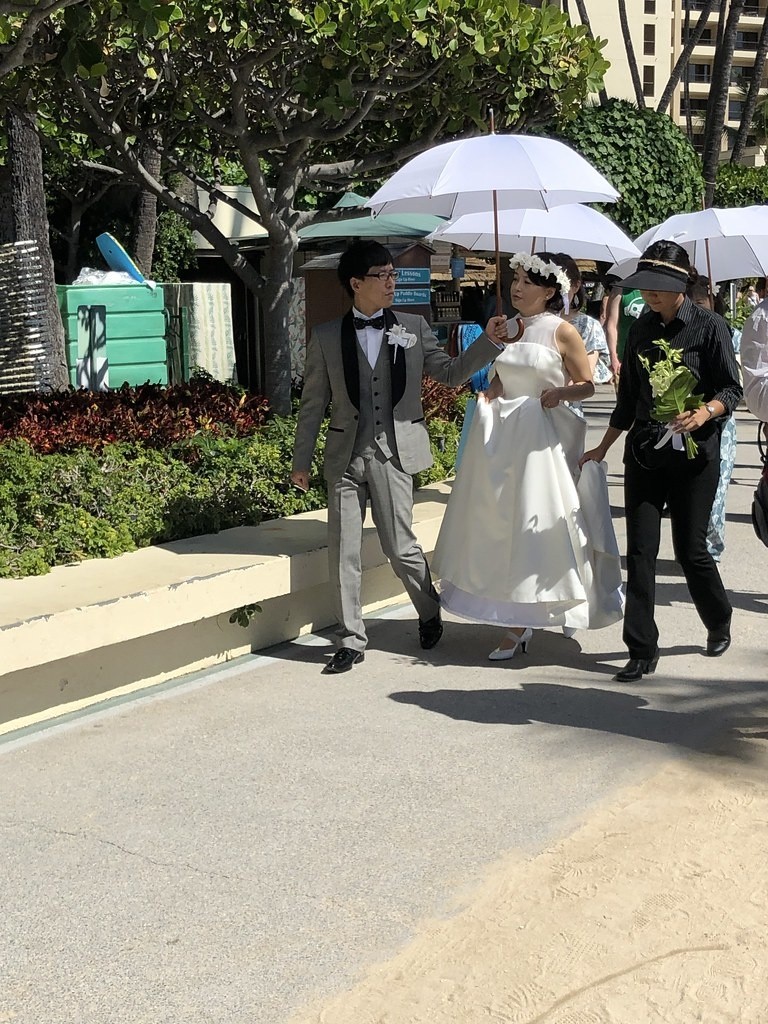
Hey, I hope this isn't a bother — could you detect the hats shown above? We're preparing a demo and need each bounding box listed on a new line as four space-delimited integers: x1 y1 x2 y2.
613 259 690 293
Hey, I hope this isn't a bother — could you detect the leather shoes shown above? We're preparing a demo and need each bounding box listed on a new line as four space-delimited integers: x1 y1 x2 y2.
419 608 443 650
326 648 364 673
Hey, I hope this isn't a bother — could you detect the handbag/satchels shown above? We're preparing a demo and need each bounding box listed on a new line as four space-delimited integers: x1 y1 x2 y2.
752 421 768 549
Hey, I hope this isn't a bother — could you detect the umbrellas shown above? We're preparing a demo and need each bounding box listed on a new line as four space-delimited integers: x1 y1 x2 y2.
362 106 624 346
427 202 766 286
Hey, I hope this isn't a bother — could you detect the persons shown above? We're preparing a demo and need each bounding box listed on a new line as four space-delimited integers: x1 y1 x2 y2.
290 240 507 675
529 252 624 630
579 238 742 685
599 280 767 570
442 251 595 661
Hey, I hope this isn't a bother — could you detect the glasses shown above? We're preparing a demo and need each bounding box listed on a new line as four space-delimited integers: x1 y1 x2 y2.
364 271 400 281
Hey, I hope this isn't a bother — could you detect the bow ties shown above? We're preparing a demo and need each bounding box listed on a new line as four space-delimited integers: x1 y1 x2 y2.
354 315 384 330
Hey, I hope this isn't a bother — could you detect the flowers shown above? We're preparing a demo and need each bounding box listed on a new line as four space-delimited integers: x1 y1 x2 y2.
638 338 706 459
509 252 571 297
385 324 410 365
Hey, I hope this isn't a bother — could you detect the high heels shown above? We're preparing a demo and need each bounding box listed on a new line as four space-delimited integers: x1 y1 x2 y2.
489 628 533 661
707 616 731 657
562 626 576 638
618 647 660 682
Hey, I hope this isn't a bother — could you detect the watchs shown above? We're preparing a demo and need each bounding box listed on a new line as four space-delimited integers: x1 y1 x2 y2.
704 404 715 421
